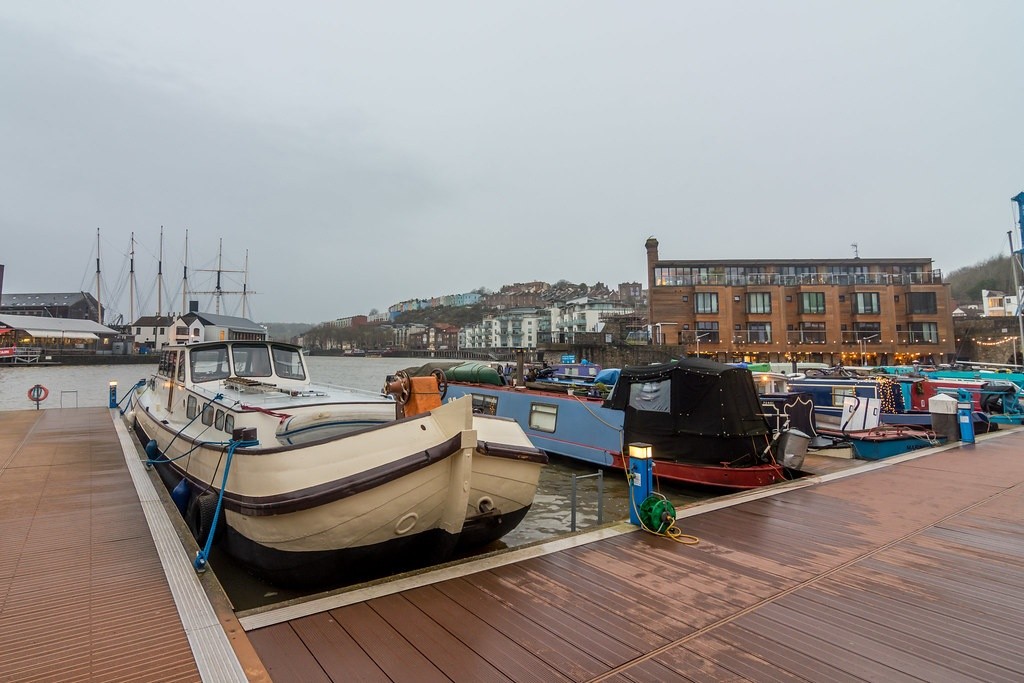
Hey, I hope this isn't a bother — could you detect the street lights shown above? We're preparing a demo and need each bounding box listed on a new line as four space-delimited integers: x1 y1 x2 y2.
695 332 710 359
863 334 880 365
656 322 678 345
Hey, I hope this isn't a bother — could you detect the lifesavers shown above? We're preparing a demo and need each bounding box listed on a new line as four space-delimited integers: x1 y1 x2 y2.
27 384 50 401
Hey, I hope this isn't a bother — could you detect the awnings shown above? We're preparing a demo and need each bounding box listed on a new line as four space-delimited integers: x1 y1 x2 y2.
24 329 100 339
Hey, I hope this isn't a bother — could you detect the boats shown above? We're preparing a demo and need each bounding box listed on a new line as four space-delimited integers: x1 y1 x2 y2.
751 354 1024 408
129 337 549 585
383 347 812 491
751 369 948 460
788 376 998 434
